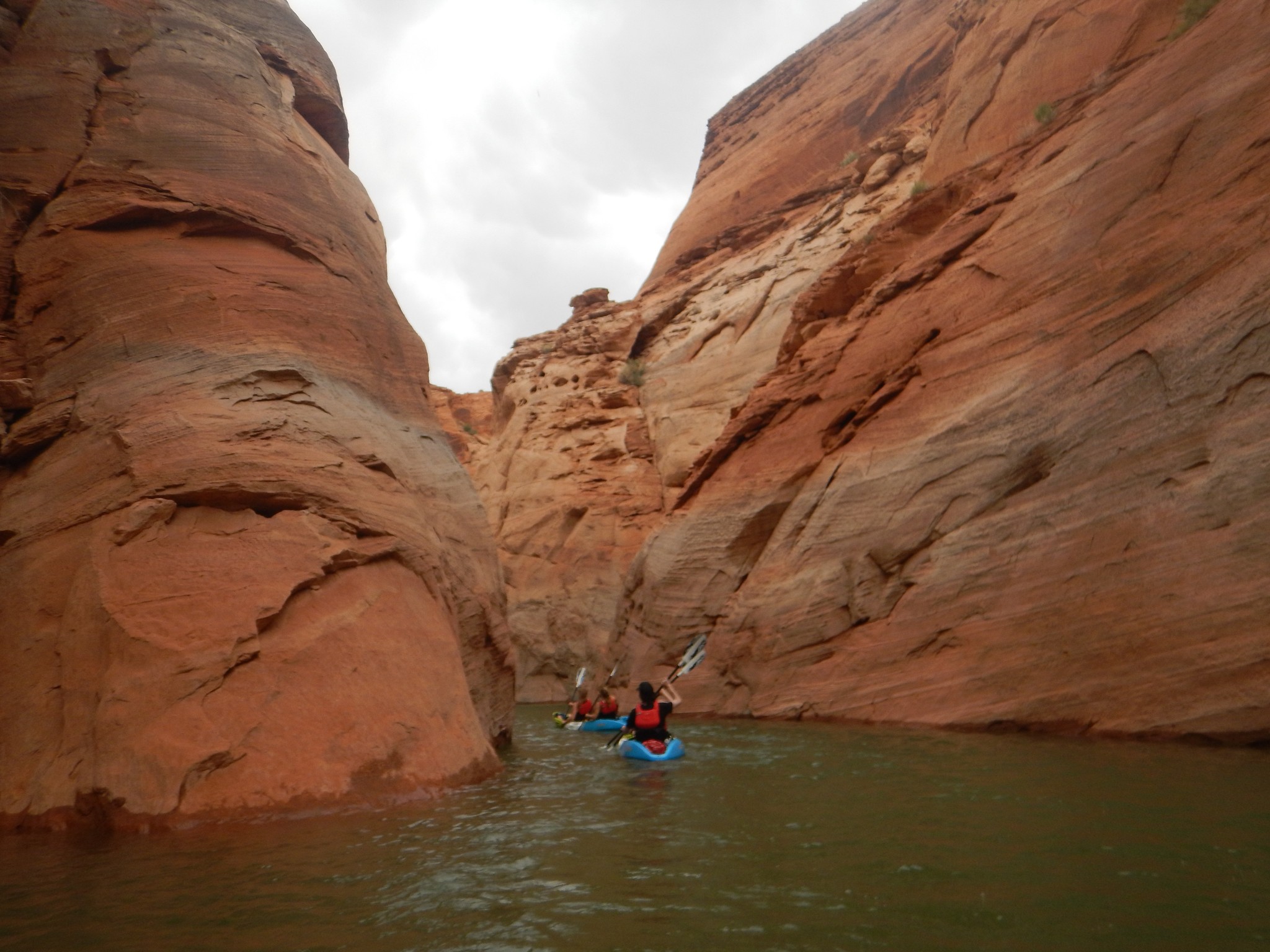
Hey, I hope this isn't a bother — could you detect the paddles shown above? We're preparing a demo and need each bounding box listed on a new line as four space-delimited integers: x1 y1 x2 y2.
606 650 705 751
600 632 708 750
572 658 623 731
564 666 586 716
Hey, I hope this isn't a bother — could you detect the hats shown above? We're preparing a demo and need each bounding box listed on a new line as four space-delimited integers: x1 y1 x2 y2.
635 682 653 692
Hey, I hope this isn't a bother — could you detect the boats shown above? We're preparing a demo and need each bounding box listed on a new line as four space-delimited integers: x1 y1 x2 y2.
617 733 684 761
552 713 630 732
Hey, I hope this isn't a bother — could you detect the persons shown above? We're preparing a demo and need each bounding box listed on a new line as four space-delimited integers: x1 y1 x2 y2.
620 677 683 743
585 687 618 721
563 689 592 722
